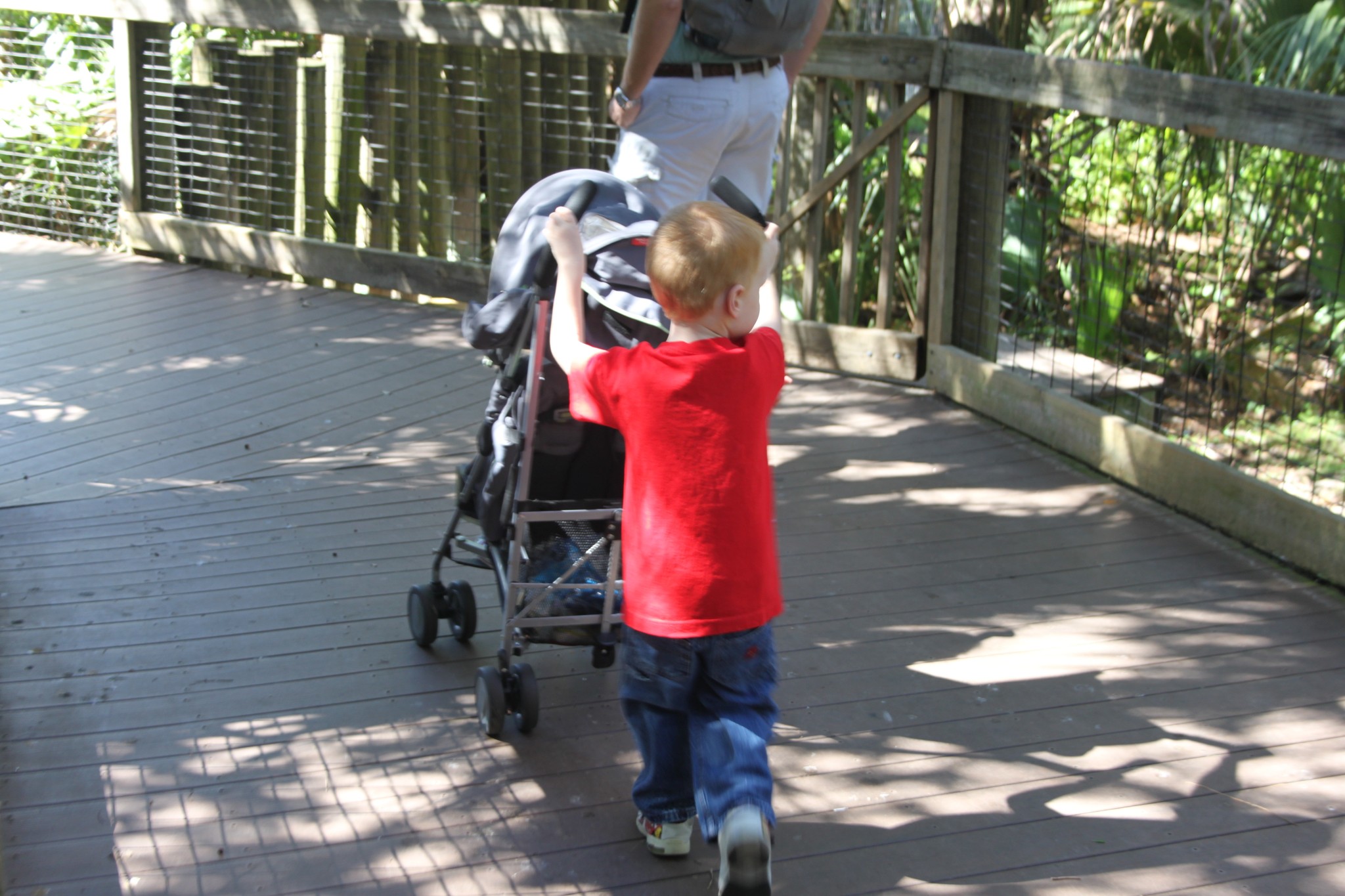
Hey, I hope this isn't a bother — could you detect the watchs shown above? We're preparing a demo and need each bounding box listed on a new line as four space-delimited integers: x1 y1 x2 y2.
614 87 641 112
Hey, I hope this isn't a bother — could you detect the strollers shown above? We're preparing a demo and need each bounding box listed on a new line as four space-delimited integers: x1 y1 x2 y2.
407 175 789 732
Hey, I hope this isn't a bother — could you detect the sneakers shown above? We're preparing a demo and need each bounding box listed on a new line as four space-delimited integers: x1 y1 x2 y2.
715 803 774 896
637 809 694 854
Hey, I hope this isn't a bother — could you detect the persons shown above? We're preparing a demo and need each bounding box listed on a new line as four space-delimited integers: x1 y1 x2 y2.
607 0 833 216
542 201 786 896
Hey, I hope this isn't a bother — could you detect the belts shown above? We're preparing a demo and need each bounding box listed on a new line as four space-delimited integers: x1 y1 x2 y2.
656 56 780 78
